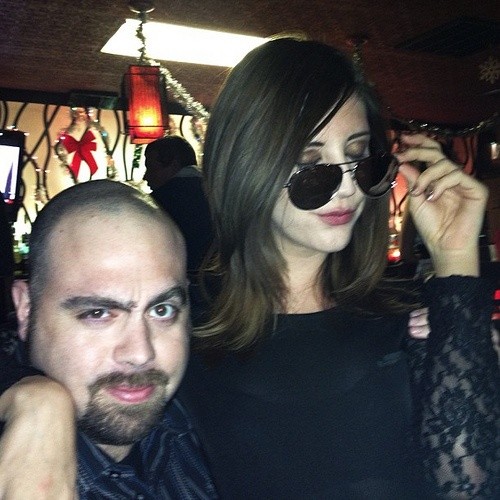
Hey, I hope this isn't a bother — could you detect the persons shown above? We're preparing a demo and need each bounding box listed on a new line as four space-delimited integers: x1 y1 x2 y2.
398 141 493 280
141 135 222 314
173 38 500 500
0 178 500 500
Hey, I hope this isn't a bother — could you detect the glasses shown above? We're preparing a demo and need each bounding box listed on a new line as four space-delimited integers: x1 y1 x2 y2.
282 154 400 210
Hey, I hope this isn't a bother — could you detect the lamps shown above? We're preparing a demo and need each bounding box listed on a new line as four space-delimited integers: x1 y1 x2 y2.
119 0 170 147
